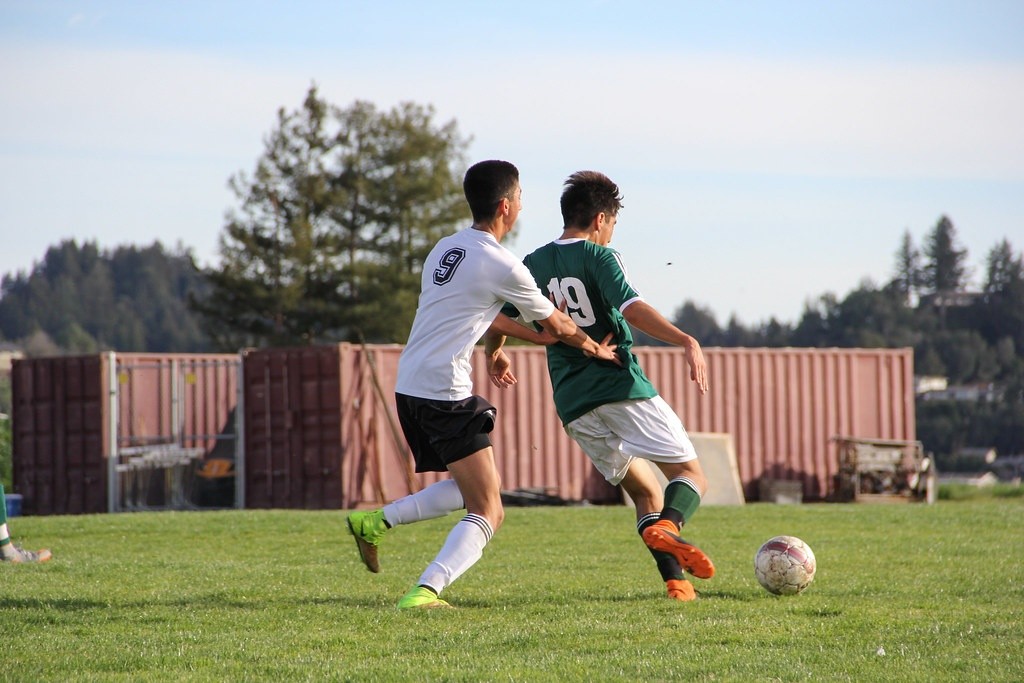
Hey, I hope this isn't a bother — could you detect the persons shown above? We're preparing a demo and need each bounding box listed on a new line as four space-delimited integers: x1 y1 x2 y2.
0 484 52 564
346 161 621 609
482 170 713 600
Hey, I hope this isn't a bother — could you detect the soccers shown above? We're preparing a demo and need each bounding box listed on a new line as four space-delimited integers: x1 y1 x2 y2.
753 534 817 596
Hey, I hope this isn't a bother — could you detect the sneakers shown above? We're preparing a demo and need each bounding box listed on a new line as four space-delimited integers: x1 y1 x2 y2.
344 508 390 573
397 585 450 612
664 578 695 602
4 547 52 564
642 519 713 579
